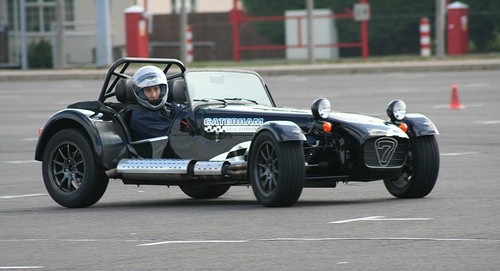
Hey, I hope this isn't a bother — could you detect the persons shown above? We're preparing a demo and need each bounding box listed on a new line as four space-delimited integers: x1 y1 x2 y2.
128 65 188 159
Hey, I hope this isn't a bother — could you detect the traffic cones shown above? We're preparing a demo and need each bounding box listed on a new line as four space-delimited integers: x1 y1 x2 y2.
449 85 462 109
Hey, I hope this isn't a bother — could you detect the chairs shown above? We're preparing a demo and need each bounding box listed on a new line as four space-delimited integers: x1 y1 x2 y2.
166 78 187 103
115 78 137 129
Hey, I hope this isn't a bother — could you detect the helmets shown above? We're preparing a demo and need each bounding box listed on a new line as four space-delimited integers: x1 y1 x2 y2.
133 65 169 110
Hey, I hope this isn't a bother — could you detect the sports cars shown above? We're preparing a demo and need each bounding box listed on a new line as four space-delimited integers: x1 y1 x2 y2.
34 57 440 208
